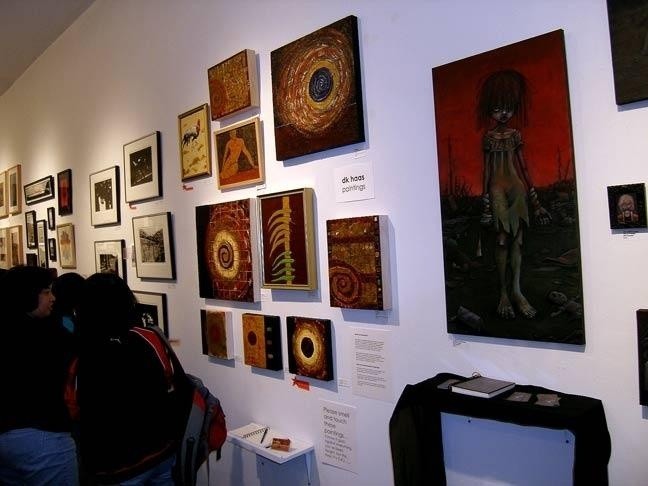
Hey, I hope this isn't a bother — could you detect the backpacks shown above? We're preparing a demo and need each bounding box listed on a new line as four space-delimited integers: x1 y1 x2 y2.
144 323 228 486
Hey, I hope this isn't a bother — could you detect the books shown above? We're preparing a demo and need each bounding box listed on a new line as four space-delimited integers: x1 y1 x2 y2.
447 375 516 399
230 422 292 449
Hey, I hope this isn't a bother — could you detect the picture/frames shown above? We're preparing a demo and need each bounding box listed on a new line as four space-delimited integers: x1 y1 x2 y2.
1 129 177 342
213 116 264 189
255 186 317 292
176 102 211 181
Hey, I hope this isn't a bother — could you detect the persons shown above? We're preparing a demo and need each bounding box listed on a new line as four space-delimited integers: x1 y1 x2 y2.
470 68 551 322
219 128 258 183
616 195 638 224
0 261 191 486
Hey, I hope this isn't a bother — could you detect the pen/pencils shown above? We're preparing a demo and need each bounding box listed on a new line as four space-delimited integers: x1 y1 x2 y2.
261 429 268 444
